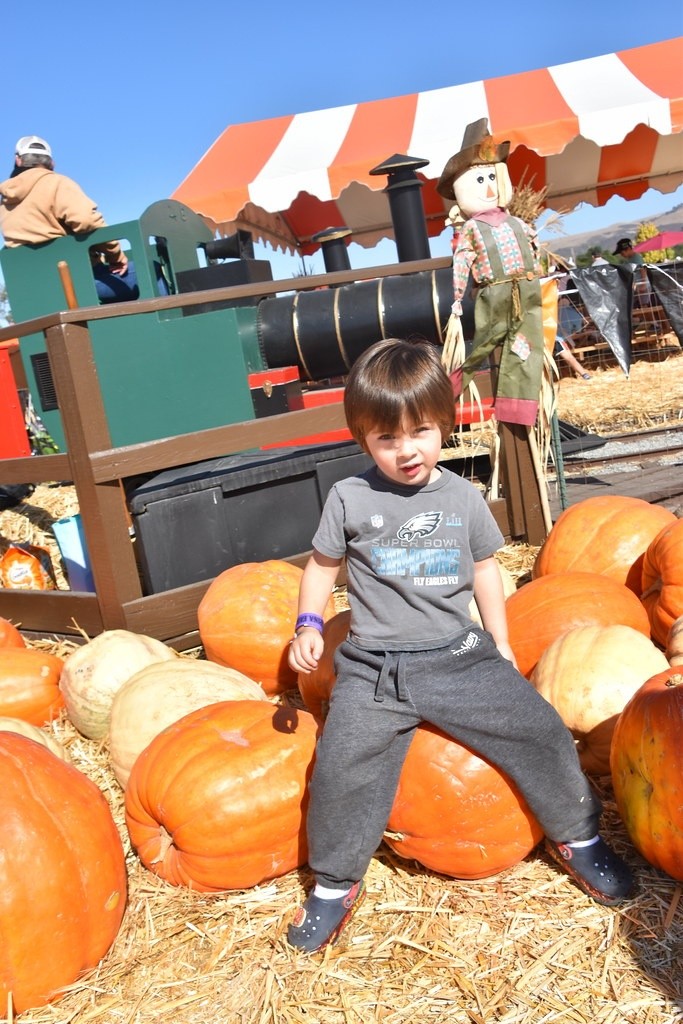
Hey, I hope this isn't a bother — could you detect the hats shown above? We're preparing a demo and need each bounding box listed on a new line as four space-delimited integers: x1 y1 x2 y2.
436 115 510 200
612 239 632 255
16 137 50 155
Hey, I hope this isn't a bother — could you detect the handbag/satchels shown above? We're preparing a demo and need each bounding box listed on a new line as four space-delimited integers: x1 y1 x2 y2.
52 513 97 592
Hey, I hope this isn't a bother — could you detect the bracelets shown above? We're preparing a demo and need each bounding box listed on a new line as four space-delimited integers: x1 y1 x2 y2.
294 612 325 634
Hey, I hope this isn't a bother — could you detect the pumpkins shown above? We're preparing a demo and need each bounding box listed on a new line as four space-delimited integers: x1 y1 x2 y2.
384 495 683 881
0 544 353 1017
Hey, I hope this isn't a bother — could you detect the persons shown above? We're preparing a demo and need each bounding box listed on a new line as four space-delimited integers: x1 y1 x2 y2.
436 117 545 428
0 135 173 306
287 338 640 954
552 238 661 379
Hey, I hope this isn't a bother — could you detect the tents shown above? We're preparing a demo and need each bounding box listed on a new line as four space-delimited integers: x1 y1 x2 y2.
168 32 683 276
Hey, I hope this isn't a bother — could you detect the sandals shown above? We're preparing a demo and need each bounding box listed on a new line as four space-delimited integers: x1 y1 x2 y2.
546 836 631 906
289 880 369 953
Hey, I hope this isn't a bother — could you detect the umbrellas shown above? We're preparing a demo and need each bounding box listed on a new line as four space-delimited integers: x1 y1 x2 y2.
632 230 683 259
